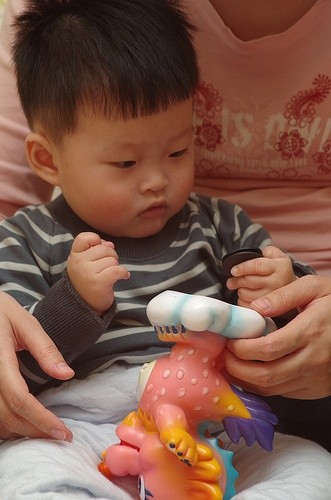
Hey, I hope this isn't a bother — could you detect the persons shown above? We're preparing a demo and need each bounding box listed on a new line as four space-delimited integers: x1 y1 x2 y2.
0 0 331 443
0 0 330 500
